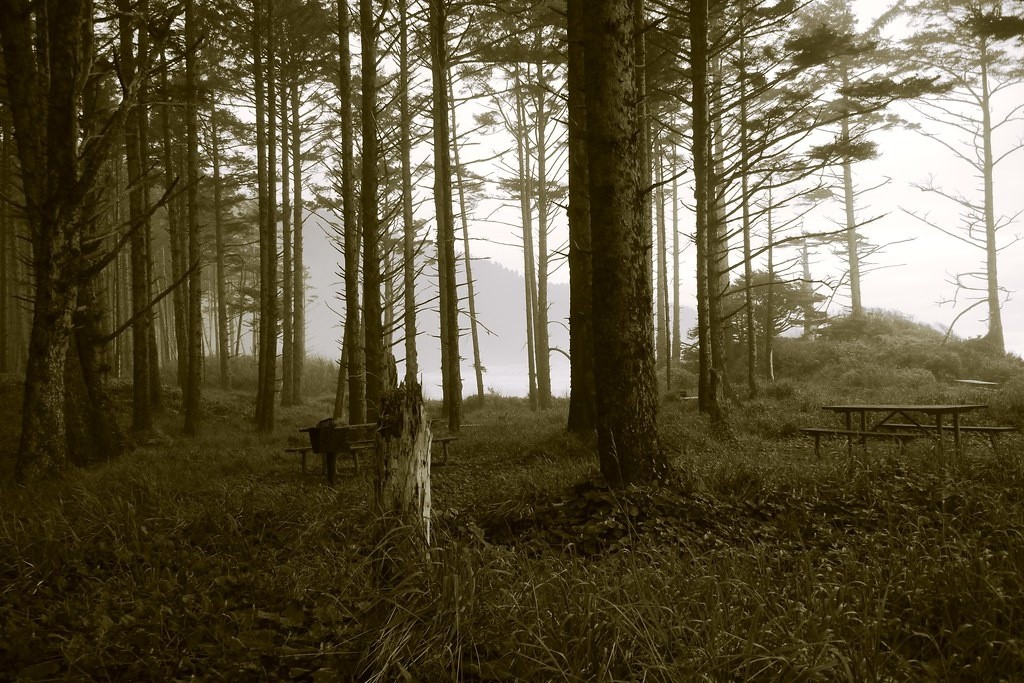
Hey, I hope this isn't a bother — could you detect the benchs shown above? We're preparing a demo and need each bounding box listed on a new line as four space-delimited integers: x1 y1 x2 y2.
878 424 1019 458
798 427 939 461
286 445 377 476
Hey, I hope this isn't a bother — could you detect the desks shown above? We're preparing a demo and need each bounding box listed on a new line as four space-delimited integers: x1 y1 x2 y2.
822 405 988 455
299 423 380 473
351 437 458 467
954 379 1000 394
